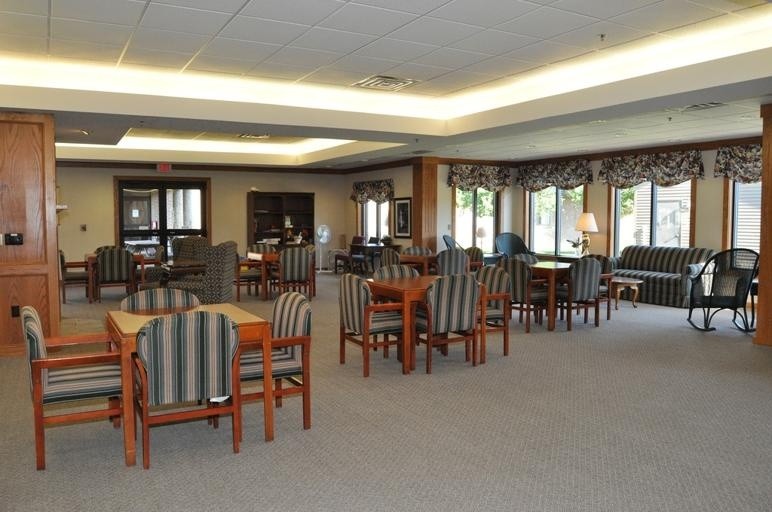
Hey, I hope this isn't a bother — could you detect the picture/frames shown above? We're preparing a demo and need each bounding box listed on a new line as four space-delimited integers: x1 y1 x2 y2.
392 197 413 239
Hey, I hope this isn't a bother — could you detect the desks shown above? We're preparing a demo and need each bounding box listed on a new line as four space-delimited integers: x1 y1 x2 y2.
363 274 485 376
86 253 145 299
248 245 277 259
528 257 575 333
240 257 261 269
348 240 402 255
402 253 439 276
484 250 501 265
105 301 274 467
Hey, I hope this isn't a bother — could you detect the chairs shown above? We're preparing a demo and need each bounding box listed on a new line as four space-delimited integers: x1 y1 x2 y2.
684 248 760 335
352 237 381 278
474 264 510 364
87 246 125 258
411 276 477 374
121 290 197 310
581 254 612 317
379 247 400 268
87 251 135 301
131 311 245 470
403 246 432 256
375 265 422 313
334 236 363 274
509 259 550 332
553 257 600 331
59 248 93 300
268 246 315 296
235 252 260 296
435 250 471 278
338 274 405 379
512 254 541 264
494 230 532 259
445 234 469 259
137 246 166 284
238 290 313 433
169 243 237 311
172 238 213 258
16 301 139 470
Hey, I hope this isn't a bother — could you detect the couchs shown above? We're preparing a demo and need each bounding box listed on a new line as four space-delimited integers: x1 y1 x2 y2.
606 239 717 309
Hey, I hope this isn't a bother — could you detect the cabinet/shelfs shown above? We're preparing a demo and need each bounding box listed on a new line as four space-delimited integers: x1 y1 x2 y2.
244 190 318 244
0 112 67 352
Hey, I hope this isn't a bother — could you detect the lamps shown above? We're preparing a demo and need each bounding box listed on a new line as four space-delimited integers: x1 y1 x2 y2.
574 212 599 257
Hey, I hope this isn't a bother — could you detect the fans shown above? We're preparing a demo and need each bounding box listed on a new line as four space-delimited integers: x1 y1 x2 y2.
315 223 333 275
